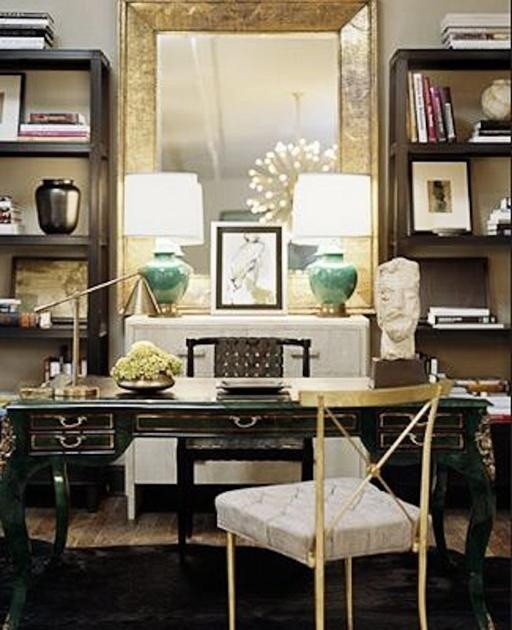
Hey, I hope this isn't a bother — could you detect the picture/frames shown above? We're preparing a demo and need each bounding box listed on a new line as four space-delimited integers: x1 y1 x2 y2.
214 227 282 310
8 255 88 326
0 71 27 137
405 155 475 236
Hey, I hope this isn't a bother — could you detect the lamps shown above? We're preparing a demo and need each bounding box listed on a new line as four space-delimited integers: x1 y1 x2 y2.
173 184 206 262
246 88 342 245
121 169 200 319
291 172 376 318
33 267 162 397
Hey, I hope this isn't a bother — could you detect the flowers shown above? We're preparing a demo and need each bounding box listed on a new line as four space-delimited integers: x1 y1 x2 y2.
108 335 184 384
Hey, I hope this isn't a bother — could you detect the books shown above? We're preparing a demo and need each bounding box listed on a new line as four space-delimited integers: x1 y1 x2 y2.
405 11 512 144
432 323 504 331
0 195 25 236
484 195 512 237
426 312 498 325
0 8 92 145
0 295 24 313
428 304 489 317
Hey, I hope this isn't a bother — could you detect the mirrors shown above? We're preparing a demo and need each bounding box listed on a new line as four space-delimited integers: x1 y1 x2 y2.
113 0 382 322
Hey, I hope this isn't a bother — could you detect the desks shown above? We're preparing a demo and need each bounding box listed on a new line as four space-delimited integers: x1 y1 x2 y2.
0 373 498 630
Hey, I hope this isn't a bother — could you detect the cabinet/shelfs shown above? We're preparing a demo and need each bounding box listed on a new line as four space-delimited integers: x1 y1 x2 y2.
124 314 374 521
0 47 112 513
382 47 512 515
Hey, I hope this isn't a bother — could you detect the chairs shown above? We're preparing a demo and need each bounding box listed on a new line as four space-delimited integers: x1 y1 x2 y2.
174 337 314 544
215 381 453 630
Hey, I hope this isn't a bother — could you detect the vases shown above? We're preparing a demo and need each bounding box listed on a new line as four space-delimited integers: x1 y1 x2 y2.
116 373 175 393
35 177 82 235
480 77 510 121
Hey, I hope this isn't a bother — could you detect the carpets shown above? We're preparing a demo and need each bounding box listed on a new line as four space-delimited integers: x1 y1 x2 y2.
0 536 512 630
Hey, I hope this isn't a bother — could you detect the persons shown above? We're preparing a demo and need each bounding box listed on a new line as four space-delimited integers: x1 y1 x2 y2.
372 257 420 365
